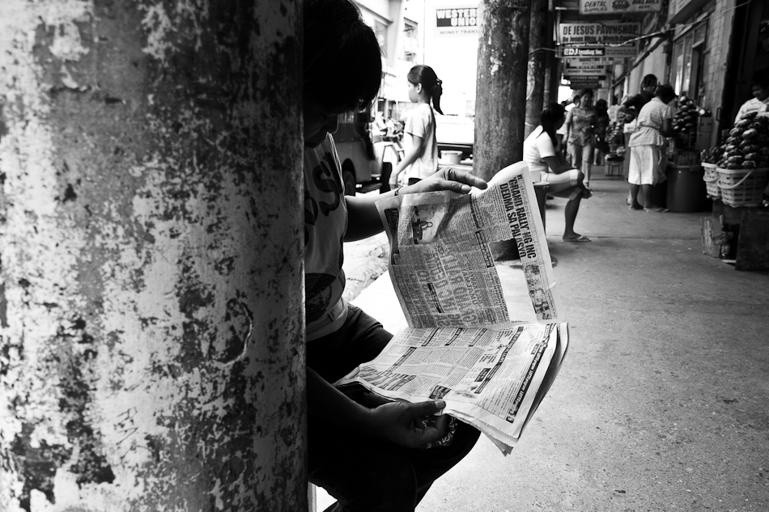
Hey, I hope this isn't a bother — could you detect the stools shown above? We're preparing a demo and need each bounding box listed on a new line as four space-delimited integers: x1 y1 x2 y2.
534 185 552 231
605 153 624 177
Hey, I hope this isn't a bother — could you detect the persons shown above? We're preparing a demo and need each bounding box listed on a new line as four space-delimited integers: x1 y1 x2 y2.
387 65 444 190
628 84 679 213
303 0 488 512
555 87 610 191
733 80 769 126
523 105 592 242
618 74 657 206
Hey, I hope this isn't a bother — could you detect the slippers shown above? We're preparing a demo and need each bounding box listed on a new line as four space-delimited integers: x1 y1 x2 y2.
563 234 591 243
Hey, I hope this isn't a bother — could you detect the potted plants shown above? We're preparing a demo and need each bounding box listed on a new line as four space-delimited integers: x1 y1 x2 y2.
668 153 710 213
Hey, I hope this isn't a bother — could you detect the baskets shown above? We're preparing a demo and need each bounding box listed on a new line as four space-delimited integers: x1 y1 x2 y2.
701 162 722 198
716 166 769 208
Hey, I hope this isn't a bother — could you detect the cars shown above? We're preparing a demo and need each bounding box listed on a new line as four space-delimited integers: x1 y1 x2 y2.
333 57 403 193
